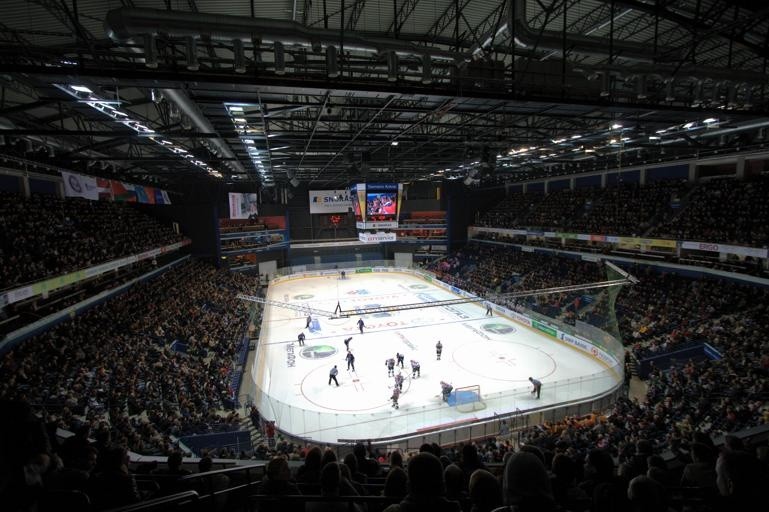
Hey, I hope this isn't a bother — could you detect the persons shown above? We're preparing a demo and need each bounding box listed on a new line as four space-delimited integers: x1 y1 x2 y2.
1 172 767 512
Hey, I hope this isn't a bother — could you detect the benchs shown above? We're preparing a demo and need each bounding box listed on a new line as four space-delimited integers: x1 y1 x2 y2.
248 475 396 512
